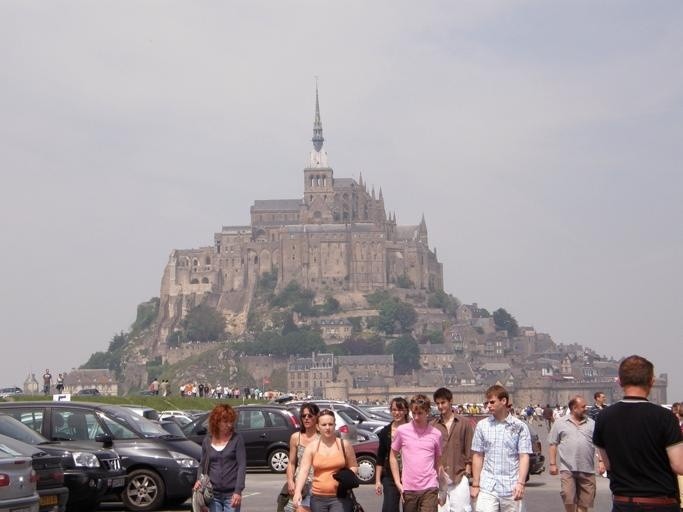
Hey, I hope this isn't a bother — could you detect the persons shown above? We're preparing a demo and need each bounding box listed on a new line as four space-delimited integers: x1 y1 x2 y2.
42 369 52 394
56 373 64 394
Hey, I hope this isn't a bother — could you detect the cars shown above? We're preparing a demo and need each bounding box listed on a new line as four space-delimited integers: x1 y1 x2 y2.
0 387 23 399
352 413 544 487
76 389 100 396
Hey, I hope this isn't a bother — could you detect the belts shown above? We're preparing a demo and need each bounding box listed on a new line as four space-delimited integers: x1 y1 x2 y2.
613 495 678 505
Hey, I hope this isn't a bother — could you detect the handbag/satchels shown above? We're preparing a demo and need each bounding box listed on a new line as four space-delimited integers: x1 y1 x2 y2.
192 474 214 512
352 502 364 512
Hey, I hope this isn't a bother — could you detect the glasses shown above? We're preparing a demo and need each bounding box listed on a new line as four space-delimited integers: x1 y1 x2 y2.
410 399 427 405
485 400 496 405
302 414 316 418
